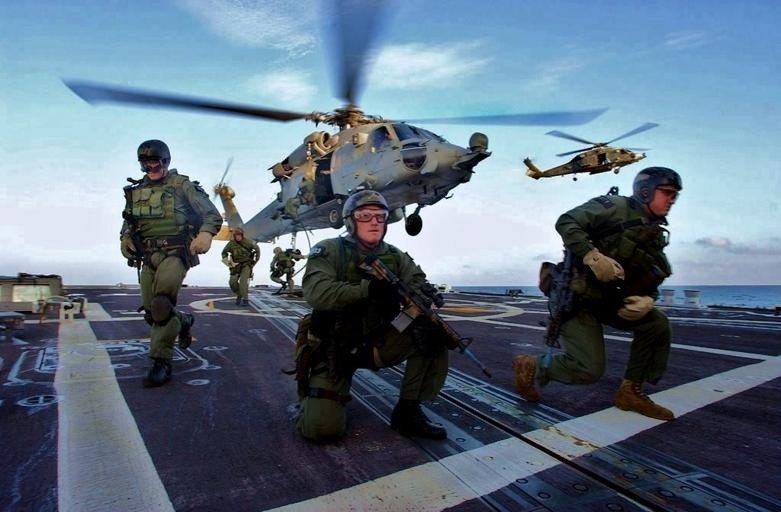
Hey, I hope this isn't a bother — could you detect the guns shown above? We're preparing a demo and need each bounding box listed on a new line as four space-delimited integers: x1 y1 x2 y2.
542 187 619 368
360 253 493 380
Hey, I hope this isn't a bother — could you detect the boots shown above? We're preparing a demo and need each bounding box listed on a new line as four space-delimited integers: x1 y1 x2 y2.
511 352 541 402
613 378 674 422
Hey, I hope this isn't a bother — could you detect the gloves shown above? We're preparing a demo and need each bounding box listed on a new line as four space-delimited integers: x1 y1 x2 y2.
189 231 213 257
617 295 655 321
120 233 137 259
580 247 626 284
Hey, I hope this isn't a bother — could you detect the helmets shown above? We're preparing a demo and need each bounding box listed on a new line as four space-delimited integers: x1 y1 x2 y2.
232 228 245 240
136 139 172 173
273 247 282 255
632 166 683 204
342 188 390 235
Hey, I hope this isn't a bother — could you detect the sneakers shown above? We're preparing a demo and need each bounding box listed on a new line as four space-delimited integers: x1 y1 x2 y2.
235 294 243 303
177 312 195 350
389 401 448 440
241 299 250 306
140 354 174 388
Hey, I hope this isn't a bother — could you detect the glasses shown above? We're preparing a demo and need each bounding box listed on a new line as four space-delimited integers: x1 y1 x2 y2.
351 207 389 224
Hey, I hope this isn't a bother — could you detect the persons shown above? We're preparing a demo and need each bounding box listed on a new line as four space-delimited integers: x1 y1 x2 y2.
283 172 315 218
120 139 222 388
285 190 449 444
513 167 683 423
221 227 260 307
270 247 304 291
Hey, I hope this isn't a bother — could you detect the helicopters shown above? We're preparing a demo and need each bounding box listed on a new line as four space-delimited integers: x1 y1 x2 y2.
522 121 660 181
62 0 609 244
